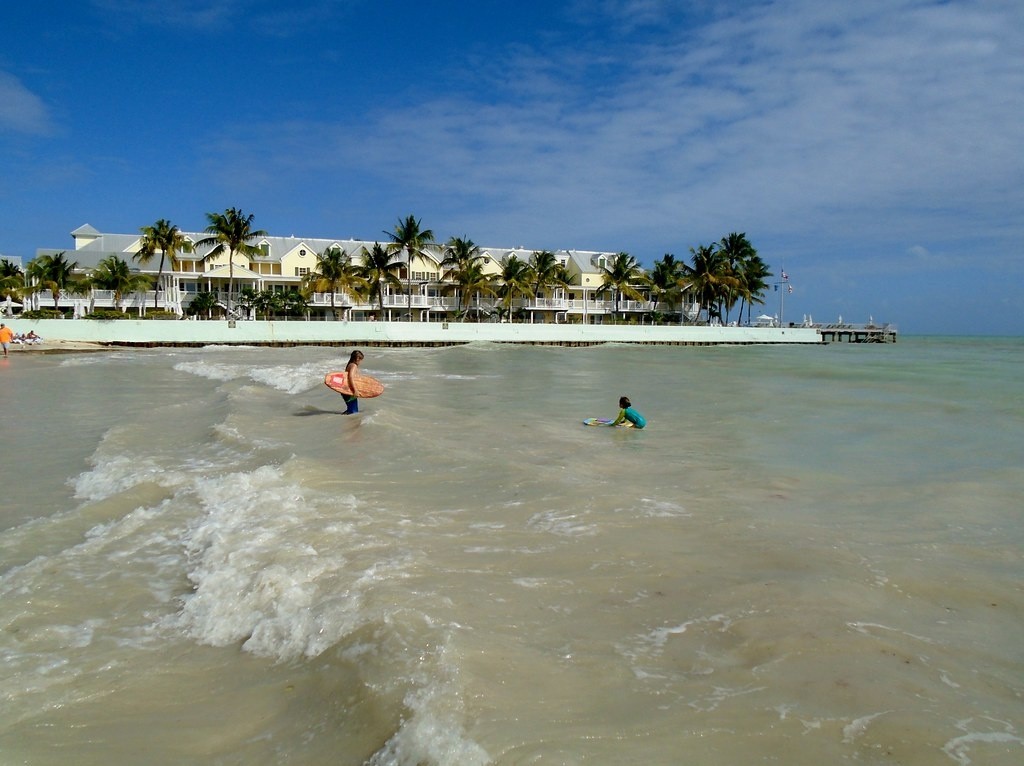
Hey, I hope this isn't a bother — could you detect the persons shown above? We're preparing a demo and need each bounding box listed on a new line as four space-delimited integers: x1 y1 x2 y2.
10 330 43 349
340 349 364 416
0 323 14 356
608 396 647 429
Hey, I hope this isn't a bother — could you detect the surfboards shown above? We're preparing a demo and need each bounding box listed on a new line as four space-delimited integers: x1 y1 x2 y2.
325 371 384 398
583 417 648 430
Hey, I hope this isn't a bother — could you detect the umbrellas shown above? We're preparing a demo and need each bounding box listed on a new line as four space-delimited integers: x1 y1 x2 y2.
757 314 873 327
